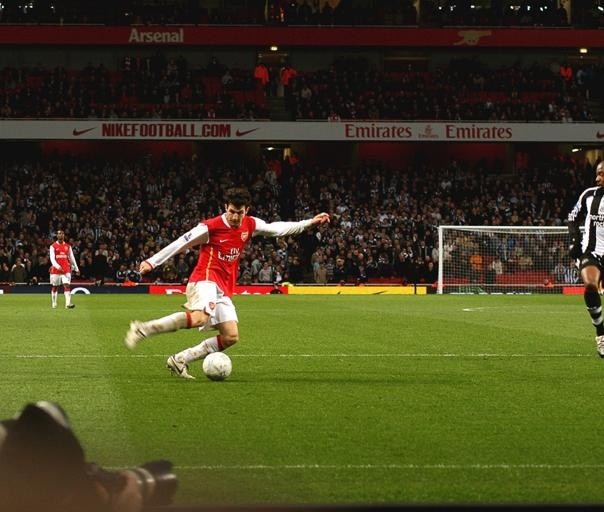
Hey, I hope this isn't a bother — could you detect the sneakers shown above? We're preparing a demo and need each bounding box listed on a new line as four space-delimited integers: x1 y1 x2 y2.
65 303 75 309
594 335 604 357
167 354 196 382
125 320 145 350
52 301 58 309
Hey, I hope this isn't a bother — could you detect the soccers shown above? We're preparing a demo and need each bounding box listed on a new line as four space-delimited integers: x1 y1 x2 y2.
202 352 232 380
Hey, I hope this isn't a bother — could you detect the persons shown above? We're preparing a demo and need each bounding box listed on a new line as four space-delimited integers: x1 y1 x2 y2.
567 164 604 359
48 230 80 308
0 418 143 512
0 0 604 281
126 185 330 381
